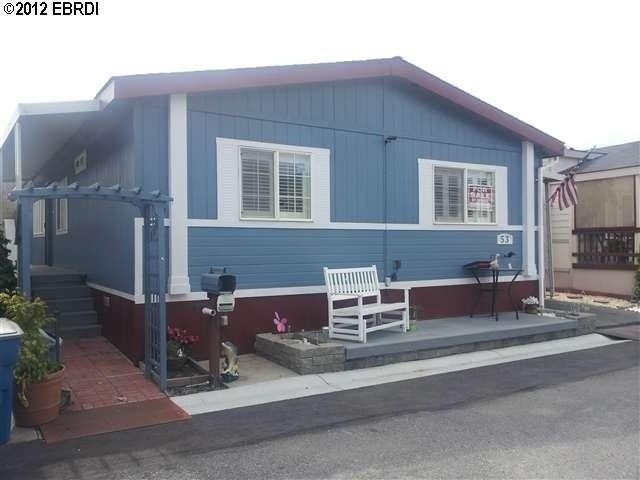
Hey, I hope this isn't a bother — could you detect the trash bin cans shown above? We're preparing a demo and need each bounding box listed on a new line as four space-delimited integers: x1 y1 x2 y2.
0 317 23 442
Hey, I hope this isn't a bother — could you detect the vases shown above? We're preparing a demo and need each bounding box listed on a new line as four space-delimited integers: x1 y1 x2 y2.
169 357 186 368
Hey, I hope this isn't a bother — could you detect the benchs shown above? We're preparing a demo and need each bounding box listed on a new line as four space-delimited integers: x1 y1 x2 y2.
323 265 409 344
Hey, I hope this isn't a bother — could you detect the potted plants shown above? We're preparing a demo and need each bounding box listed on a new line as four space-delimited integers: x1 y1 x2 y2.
521 296 540 314
0 288 66 428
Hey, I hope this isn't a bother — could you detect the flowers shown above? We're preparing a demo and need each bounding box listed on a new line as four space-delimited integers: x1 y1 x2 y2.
167 326 199 355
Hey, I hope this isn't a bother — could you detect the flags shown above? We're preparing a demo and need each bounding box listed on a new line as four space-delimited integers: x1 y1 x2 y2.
550 151 593 212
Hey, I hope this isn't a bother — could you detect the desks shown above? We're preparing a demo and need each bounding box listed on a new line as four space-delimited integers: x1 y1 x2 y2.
470 268 523 322
